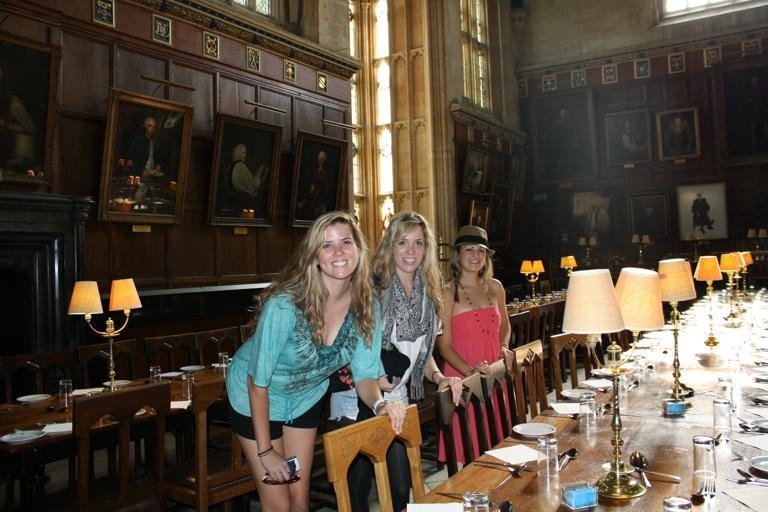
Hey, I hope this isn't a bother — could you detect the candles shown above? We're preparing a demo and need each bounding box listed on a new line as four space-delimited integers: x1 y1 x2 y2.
745 228 756 238
757 228 768 238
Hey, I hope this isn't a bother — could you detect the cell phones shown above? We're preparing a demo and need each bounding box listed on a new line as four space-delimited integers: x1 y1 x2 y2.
285 456 300 472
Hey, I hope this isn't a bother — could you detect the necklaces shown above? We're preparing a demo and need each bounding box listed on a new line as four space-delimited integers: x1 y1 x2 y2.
457 277 496 333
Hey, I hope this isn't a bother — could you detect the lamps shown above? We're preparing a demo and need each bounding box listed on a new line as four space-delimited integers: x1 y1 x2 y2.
518 258 544 299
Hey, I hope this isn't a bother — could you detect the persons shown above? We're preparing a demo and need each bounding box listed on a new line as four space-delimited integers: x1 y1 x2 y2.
222 209 406 512
300 150 327 218
618 118 645 160
127 116 167 201
691 191 714 234
226 144 265 210
318 208 464 512
549 104 589 174
586 198 608 231
437 224 513 467
667 117 692 154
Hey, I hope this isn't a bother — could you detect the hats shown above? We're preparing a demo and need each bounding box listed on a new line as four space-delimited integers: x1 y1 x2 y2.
449 226 495 256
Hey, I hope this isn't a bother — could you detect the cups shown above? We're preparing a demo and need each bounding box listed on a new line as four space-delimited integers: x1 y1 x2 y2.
537 478 561 510
692 436 717 479
712 398 732 431
718 376 732 403
513 289 566 313
462 491 490 512
662 495 693 512
535 437 560 479
580 391 596 425
218 351 229 376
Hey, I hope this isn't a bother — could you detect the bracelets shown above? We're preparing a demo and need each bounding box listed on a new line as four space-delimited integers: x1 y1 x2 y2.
257 446 273 457
372 399 387 418
432 370 439 382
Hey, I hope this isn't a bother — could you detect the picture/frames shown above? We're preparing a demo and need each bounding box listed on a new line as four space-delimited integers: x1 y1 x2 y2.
286 123 354 232
0 28 68 192
202 109 284 233
91 85 197 230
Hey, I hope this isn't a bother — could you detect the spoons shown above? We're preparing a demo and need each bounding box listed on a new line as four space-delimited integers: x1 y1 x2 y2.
474 457 524 479
558 447 580 475
737 467 757 482
437 490 513 512
643 364 654 375
597 402 613 420
627 379 640 392
630 449 654 490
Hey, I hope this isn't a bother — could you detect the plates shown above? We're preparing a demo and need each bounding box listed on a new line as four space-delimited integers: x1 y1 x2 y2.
512 421 556 439
0 365 205 445
591 368 615 378
741 346 768 475
561 388 596 401
